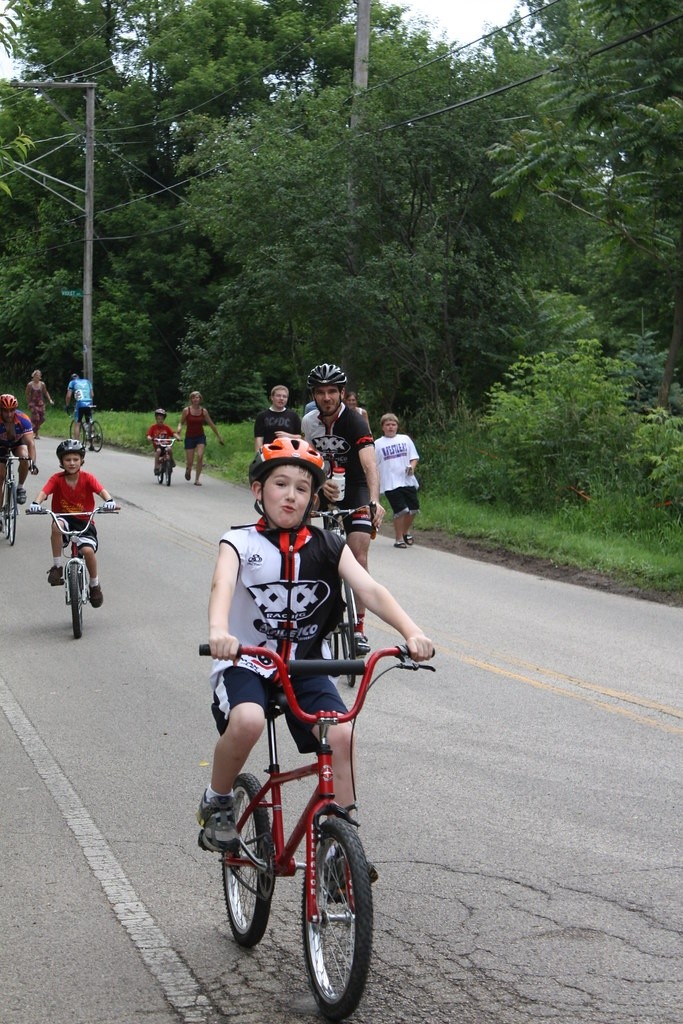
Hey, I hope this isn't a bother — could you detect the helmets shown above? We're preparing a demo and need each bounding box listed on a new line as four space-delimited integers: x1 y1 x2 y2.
56 439 86 462
306 363 348 391
0 394 18 409
249 436 326 493
155 408 166 419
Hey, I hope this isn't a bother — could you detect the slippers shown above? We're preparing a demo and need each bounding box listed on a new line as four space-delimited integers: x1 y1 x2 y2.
395 542 407 548
403 533 414 545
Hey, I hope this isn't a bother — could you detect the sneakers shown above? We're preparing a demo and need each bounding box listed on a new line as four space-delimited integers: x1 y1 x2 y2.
321 846 379 905
46 565 63 584
89 582 104 608
196 787 241 853
353 635 372 655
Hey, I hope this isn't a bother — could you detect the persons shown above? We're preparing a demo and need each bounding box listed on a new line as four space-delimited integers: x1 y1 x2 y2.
304 400 317 414
346 392 372 434
373 413 421 548
0 394 39 531
253 385 302 452
29 439 115 608
146 409 182 475
25 370 54 439
195 438 434 902
65 373 94 451
177 391 224 486
301 362 387 654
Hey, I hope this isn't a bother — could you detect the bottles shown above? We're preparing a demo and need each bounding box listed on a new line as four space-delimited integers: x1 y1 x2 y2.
331 467 346 502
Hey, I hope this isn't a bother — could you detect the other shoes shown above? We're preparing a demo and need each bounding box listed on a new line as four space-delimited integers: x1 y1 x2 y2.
170 459 176 467
154 468 160 476
0 520 3 532
185 468 191 481
16 487 26 504
194 482 201 486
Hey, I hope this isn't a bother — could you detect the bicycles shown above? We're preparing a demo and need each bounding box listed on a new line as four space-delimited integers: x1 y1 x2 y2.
194 644 437 1023
310 504 380 687
66 403 104 453
0 448 34 546
26 503 123 639
148 434 183 486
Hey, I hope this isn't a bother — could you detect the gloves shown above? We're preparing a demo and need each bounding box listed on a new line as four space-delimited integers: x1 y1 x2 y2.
29 501 41 513
65 405 69 411
103 499 116 510
